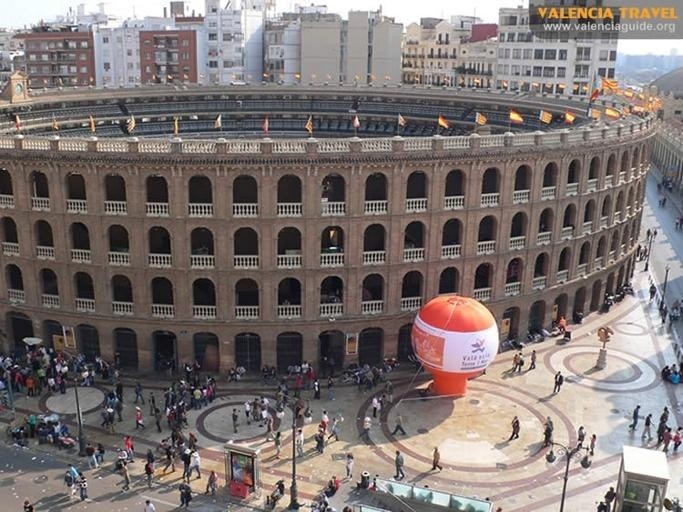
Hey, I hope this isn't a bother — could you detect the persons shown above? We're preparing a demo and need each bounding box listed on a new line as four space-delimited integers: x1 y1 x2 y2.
508 313 567 351
637 244 648 261
628 404 683 453
676 216 683 230
432 446 443 470
577 426 597 455
649 284 683 321
661 363 683 384
597 486 617 512
553 371 563 393
646 228 657 240
509 416 520 440
543 416 553 446
658 197 666 208
662 176 673 192
513 350 536 372
0 344 407 511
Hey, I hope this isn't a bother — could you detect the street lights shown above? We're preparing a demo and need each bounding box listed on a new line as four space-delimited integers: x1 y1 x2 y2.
660 263 670 310
646 228 657 270
275 391 313 509
0 364 19 411
60 356 89 457
545 440 592 512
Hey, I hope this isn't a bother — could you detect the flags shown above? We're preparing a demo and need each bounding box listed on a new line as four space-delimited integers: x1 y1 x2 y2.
14 70 662 135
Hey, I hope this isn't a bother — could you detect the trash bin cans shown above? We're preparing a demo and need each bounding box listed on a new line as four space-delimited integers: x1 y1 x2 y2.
565 331 570 340
